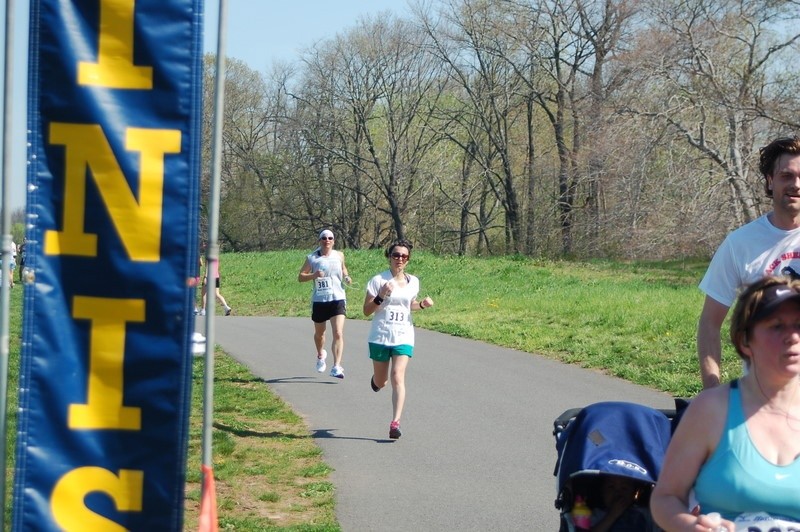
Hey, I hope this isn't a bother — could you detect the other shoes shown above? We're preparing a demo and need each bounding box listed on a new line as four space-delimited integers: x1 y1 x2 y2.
225 306 232 316
317 349 327 372
193 307 199 316
199 309 205 316
330 366 344 379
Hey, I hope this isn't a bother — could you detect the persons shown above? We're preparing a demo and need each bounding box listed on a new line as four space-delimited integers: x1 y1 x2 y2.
696 137 800 388
649 273 800 532
363 240 433 439
297 230 352 380
193 256 232 316
588 474 662 532
0 237 26 288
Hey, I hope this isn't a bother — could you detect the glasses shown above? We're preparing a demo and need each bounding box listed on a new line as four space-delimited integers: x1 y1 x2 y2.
390 253 410 262
319 236 333 241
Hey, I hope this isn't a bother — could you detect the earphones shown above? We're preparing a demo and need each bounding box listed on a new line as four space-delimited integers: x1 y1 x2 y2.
742 339 750 348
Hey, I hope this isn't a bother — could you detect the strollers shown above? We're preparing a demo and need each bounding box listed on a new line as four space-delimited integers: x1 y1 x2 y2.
552 398 692 532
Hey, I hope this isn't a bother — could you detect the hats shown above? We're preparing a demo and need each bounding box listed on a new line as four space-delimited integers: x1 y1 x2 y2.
752 283 800 320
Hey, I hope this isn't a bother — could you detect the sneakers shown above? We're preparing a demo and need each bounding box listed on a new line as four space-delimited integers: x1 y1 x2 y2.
389 421 401 439
371 375 380 392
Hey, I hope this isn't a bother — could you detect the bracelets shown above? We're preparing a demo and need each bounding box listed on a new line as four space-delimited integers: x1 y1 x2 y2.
204 276 207 278
420 301 424 309
374 295 384 305
